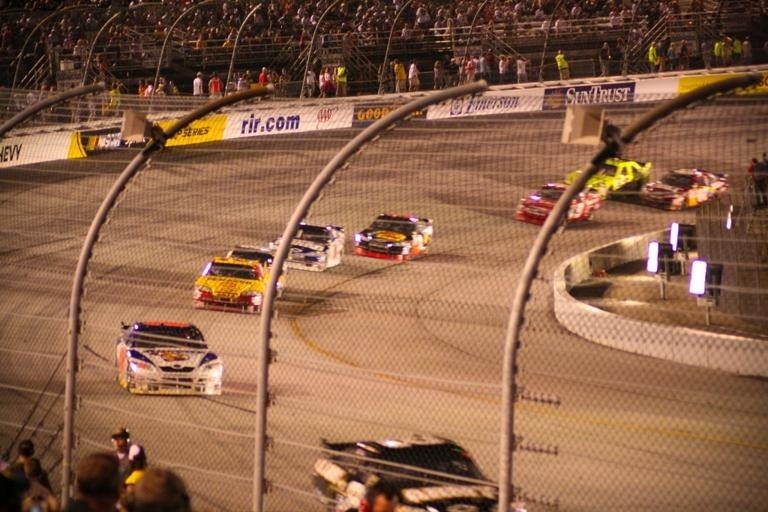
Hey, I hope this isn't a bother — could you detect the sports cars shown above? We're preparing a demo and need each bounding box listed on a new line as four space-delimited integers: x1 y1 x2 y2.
194 212 434 314
313 438 499 512
515 156 728 234
115 321 223 396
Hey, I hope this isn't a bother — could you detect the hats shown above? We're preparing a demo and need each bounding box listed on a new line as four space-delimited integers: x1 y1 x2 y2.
18 439 35 455
124 443 145 463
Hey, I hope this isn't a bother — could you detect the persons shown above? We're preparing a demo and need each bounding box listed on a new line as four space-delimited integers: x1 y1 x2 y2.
140 119 163 171
599 118 620 146
0 1 765 117
748 155 768 209
760 152 768 166
0 426 194 512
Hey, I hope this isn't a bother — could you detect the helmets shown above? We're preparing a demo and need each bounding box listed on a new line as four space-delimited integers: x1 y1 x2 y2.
111 428 128 439
